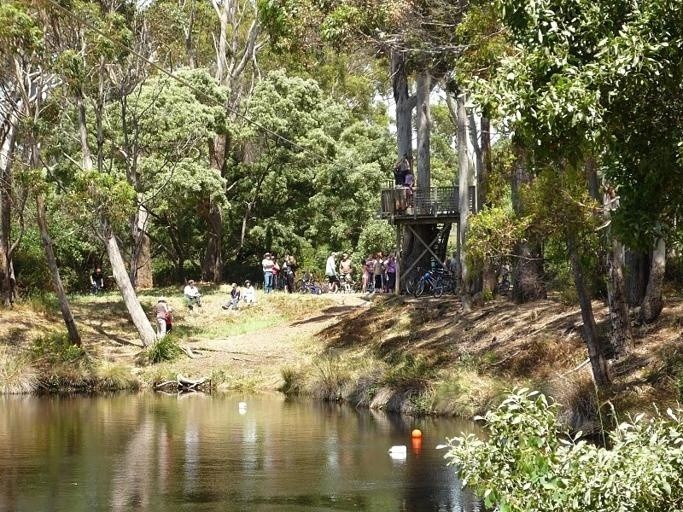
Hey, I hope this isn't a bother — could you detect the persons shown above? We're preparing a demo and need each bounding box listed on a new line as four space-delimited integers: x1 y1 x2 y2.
165 307 173 333
221 282 240 310
391 159 410 215
237 279 257 310
88 268 104 295
183 279 200 310
153 296 168 341
404 168 413 207
431 250 510 292
262 249 397 295
